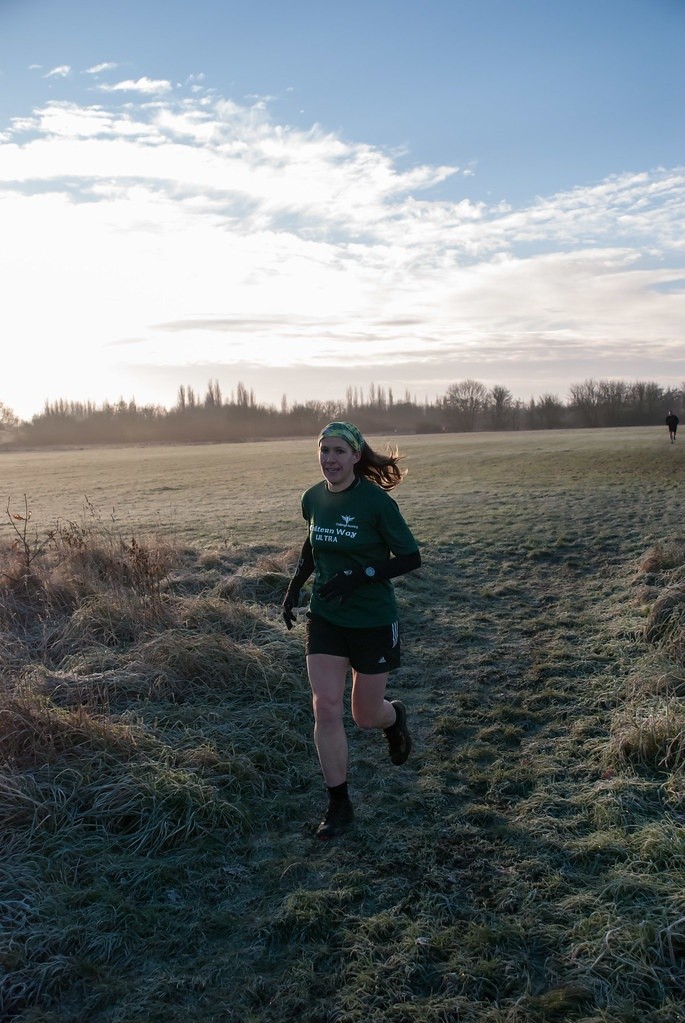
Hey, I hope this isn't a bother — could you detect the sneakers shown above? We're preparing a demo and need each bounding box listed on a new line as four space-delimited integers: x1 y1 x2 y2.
383 700 412 766
316 800 356 839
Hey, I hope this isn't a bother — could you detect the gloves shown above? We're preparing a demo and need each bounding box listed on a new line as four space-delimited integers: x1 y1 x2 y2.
316 549 421 604
282 537 315 630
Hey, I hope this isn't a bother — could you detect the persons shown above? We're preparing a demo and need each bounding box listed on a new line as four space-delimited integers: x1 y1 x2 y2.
282 422 420 839
666 411 679 443
